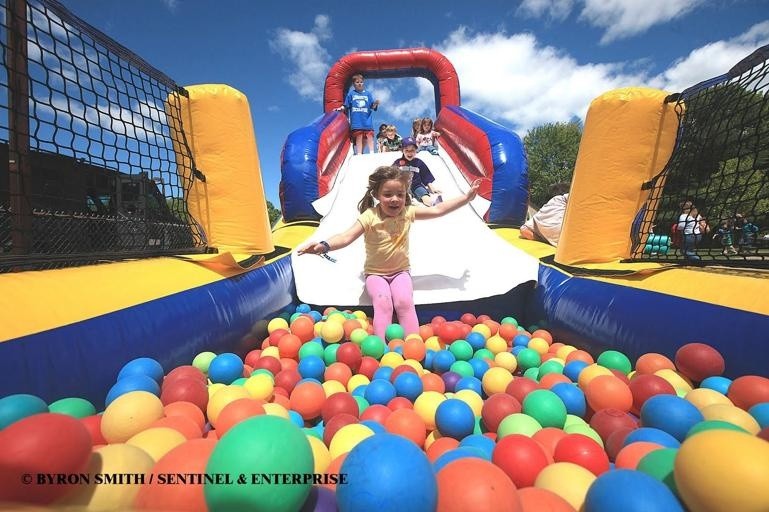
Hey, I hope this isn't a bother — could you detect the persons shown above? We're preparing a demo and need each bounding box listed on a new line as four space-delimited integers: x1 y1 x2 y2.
737 218 759 247
419 311 481 343
673 200 709 265
269 314 297 342
489 343 769 512
298 304 371 343
297 166 481 342
484 314 558 342
392 137 442 207
283 336 488 512
0 341 281 512
384 323 404 340
520 181 570 250
333 74 380 155
377 116 439 154
713 221 737 255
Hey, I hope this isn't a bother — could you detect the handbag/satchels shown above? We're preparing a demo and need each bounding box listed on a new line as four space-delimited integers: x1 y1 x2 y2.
699 217 710 233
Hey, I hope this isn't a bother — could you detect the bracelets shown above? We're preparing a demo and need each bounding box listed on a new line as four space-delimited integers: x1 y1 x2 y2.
319 241 337 263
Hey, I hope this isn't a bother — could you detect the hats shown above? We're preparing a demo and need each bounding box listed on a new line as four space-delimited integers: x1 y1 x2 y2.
401 136 419 149
